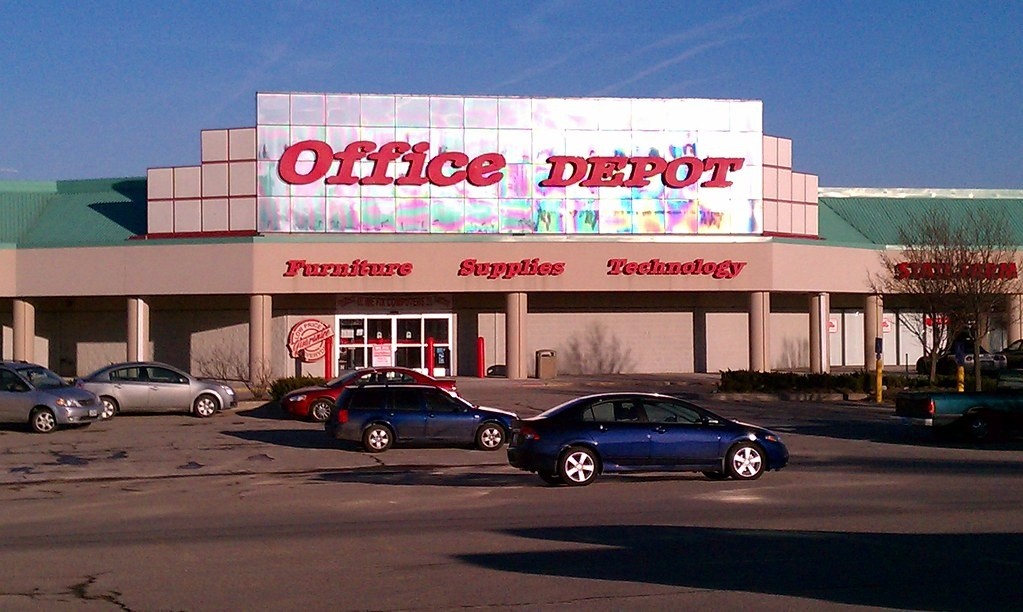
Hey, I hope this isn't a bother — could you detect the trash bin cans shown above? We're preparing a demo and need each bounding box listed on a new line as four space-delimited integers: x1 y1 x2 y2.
535 349 556 379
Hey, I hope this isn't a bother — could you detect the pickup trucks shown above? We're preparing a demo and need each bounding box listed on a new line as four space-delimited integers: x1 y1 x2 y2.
889 372 1023 440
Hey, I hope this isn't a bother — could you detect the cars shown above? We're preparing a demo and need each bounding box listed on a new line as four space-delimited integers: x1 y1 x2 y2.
0 358 103 433
506 390 791 486
277 365 459 422
996 339 1023 372
915 341 1005 376
324 386 520 449
68 361 237 417
